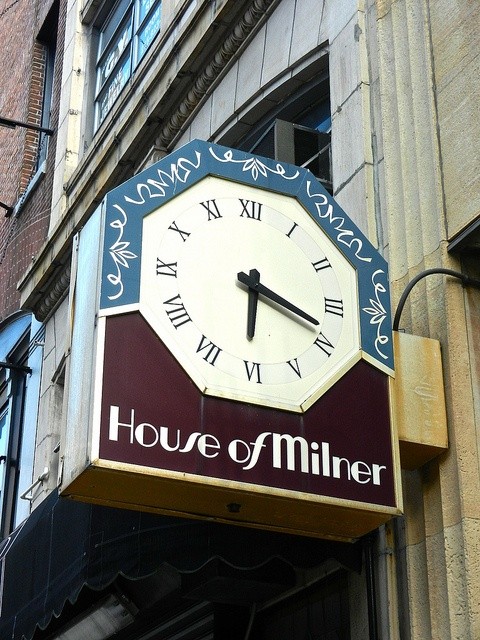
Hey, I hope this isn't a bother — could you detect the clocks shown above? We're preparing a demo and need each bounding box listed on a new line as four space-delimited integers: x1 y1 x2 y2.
57 137 407 547
